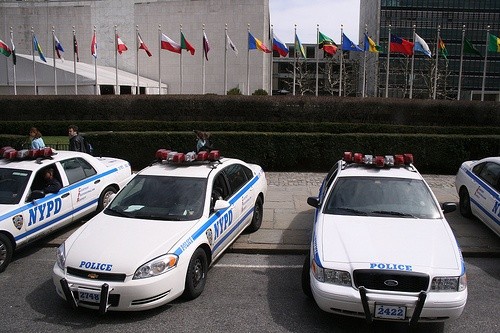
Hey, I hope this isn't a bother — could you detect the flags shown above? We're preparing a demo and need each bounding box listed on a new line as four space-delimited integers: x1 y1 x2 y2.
161 33 181 53
34 35 46 62
10 33 16 65
74 34 79 62
116 34 127 54
137 33 152 56
181 33 195 55
55 36 64 60
295 35 306 60
203 31 210 60
91 32 97 57
0 40 11 57
248 32 271 53
319 32 338 58
272 31 290 57
226 35 238 55
342 32 500 59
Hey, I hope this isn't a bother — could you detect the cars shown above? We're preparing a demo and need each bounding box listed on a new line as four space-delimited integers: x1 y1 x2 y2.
302 158 468 331
454 156 500 237
0 150 132 272
52 157 267 316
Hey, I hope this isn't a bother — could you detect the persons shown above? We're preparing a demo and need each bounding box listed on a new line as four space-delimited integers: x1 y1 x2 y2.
193 131 206 152
30 168 62 195
30 127 44 150
69 126 91 154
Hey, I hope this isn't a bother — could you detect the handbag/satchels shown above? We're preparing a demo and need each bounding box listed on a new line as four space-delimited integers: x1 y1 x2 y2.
83 140 94 155
